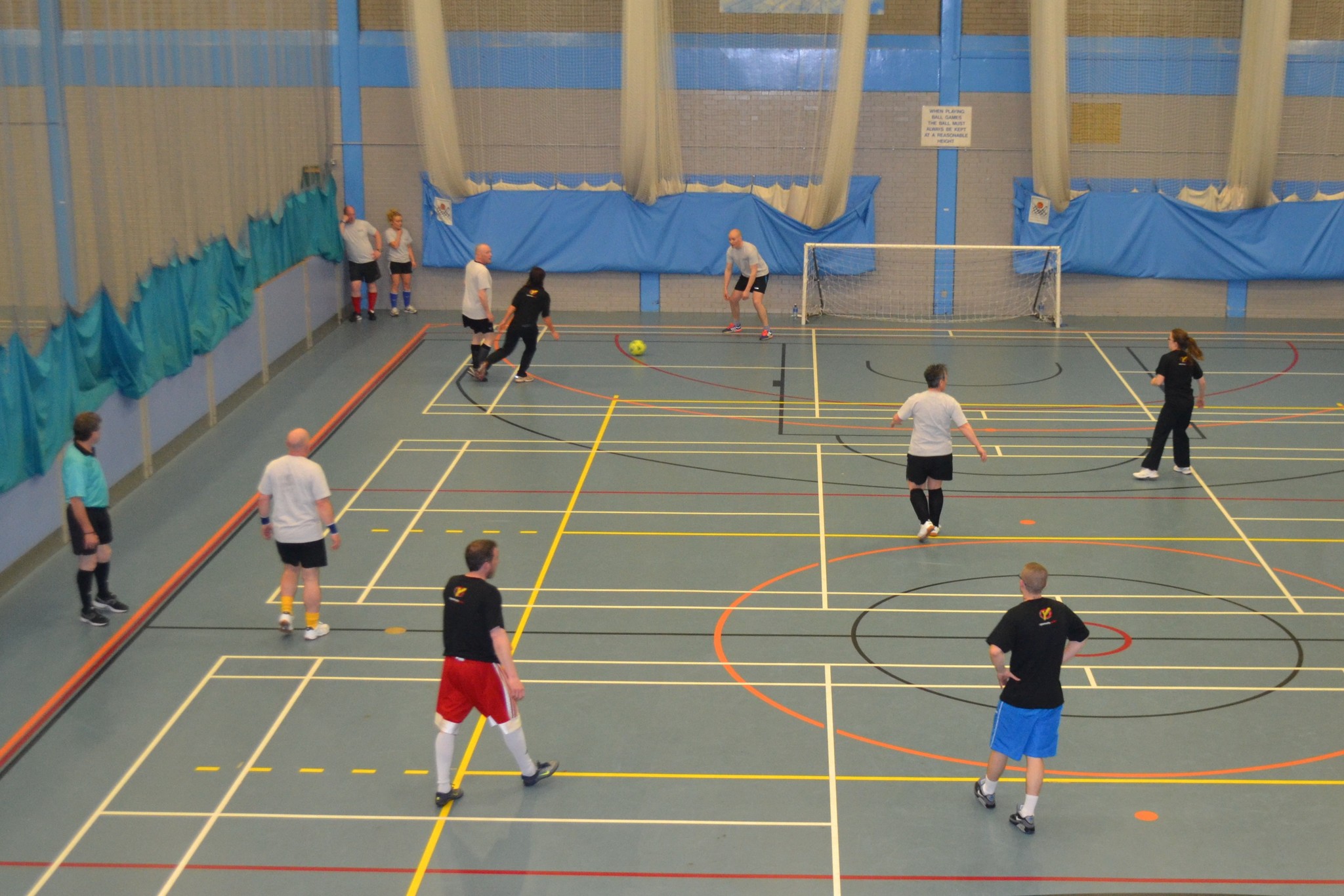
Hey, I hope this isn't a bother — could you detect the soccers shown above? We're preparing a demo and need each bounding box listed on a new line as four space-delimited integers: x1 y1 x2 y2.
629 339 647 356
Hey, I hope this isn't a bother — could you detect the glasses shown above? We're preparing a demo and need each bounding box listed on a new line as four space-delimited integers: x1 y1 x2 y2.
1018 571 1027 586
1167 338 1174 342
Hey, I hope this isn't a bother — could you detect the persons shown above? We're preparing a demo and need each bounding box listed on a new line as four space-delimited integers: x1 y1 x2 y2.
476 266 560 382
433 538 558 806
1133 329 1206 479
722 229 773 340
62 410 128 627
257 427 340 640
891 363 988 542
340 206 382 321
384 209 417 316
973 562 1090 834
462 243 496 381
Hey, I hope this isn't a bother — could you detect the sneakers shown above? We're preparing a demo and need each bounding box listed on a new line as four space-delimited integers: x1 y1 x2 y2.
1133 467 1159 480
349 312 363 322
759 330 773 340
929 524 941 537
917 520 935 541
390 308 399 316
304 621 330 640
722 324 741 333
279 612 294 635
92 592 129 612
468 367 488 382
1173 465 1191 474
80 608 110 626
435 785 463 808
404 305 417 314
515 375 534 382
1009 803 1035 834
367 311 376 320
522 760 562 787
974 777 996 809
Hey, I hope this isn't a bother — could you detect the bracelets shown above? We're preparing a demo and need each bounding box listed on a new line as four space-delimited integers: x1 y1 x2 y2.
83 531 96 534
340 220 346 224
376 248 381 252
